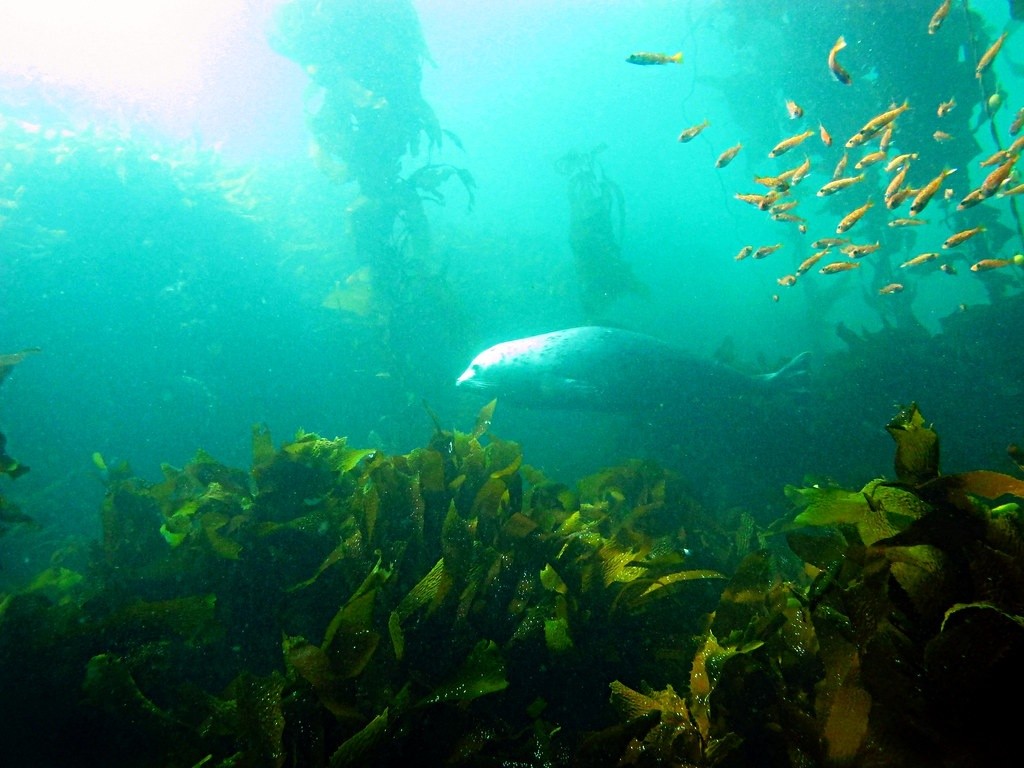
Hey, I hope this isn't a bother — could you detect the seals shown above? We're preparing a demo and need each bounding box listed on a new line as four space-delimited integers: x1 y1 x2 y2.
456 327 813 411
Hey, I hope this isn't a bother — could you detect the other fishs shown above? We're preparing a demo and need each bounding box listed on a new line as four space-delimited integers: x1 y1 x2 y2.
625 0 1024 302
